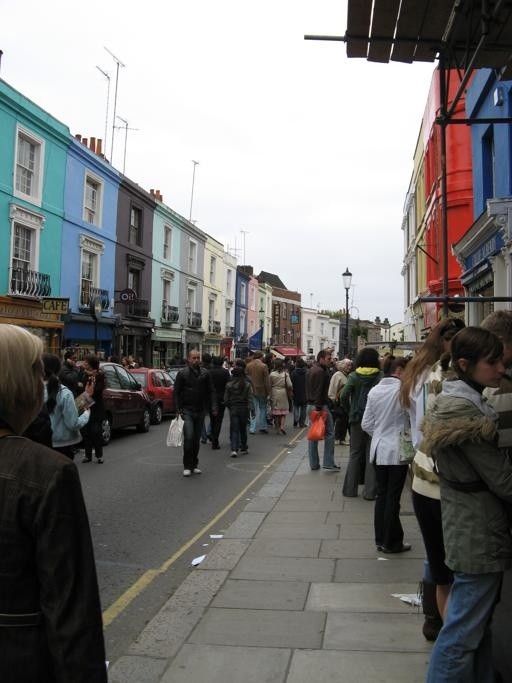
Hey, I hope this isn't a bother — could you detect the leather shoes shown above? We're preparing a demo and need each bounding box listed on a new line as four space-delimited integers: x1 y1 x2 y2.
377 540 411 553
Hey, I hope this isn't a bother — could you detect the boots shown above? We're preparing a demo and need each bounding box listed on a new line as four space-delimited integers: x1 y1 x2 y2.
416 577 443 641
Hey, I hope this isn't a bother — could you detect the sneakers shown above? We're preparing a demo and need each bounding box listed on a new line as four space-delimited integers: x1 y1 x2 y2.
333 439 350 445
230 449 248 457
259 420 299 435
97 458 104 463
183 469 191 476
82 457 91 464
193 468 201 474
201 432 221 450
323 464 341 471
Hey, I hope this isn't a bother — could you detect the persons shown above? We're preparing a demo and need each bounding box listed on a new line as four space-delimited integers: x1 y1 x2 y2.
110 356 143 370
305 348 413 554
170 348 308 475
1 324 110 683
397 311 512 682
23 350 108 465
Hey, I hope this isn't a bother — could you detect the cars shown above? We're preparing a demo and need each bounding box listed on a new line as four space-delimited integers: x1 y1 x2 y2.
97 362 152 446
127 368 182 425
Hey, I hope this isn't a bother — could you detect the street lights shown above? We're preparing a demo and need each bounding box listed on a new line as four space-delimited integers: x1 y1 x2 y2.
342 268 352 355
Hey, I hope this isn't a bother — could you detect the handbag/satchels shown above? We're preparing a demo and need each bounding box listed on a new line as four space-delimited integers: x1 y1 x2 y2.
289 399 294 412
166 415 184 447
398 430 415 462
413 437 441 485
